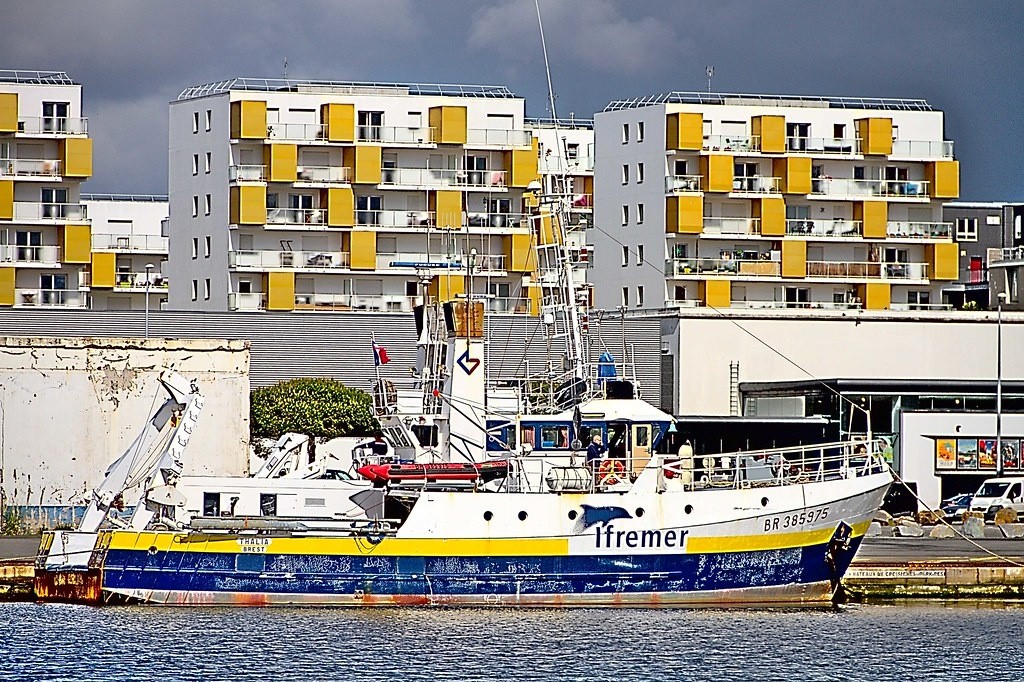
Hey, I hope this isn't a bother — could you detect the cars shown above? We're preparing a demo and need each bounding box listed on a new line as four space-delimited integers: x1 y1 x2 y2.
940 495 964 510
942 496 973 525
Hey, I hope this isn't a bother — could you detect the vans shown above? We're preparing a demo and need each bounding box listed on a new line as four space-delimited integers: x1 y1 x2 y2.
968 477 1024 522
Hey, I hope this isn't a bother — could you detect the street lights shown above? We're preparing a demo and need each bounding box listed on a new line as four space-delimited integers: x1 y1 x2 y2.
144 263 156 339
996 292 1009 478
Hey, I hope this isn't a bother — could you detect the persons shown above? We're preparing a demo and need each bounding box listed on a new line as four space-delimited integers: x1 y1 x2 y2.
587 435 605 493
354 431 388 463
678 438 697 488
308 437 316 464
853 436 894 463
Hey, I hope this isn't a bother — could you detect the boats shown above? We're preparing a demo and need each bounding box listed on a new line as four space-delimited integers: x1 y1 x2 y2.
35 1 899 614
352 459 515 494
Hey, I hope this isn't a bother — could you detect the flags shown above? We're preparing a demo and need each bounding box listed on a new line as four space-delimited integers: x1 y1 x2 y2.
370 336 392 366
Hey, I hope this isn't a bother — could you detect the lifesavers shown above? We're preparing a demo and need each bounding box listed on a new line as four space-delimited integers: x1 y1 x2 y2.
599 460 624 485
